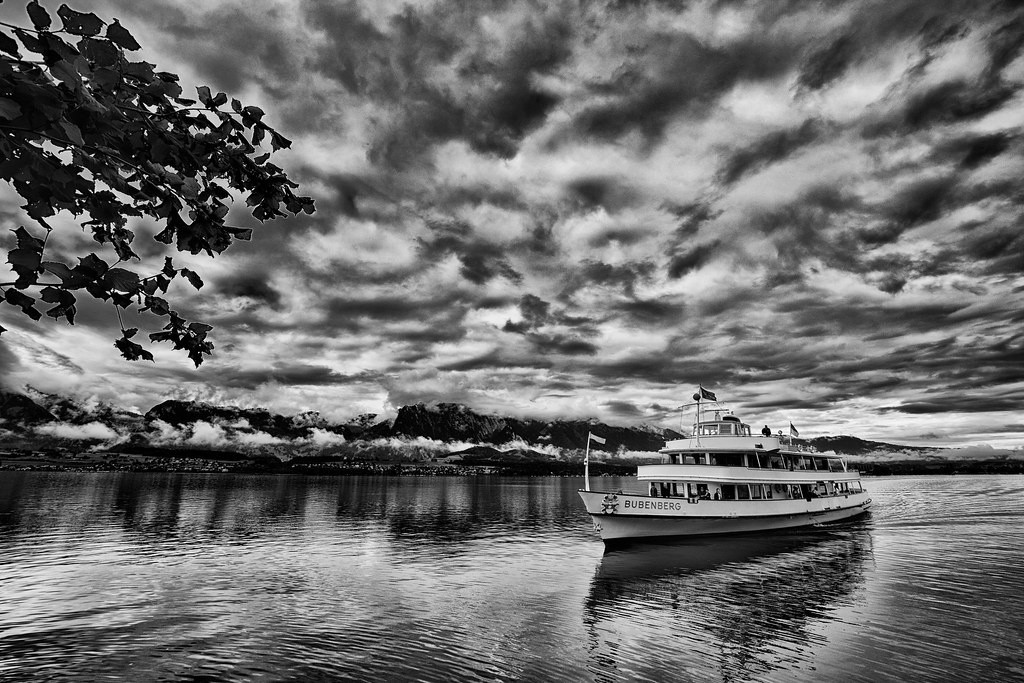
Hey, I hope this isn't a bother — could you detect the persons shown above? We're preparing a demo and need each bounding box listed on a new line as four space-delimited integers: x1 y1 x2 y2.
792 486 819 499
650 485 657 498
700 487 711 500
835 486 850 496
762 425 771 437
714 488 722 500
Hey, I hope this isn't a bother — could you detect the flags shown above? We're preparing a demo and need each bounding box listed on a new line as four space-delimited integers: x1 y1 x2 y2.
790 423 799 437
700 387 716 402
589 434 606 445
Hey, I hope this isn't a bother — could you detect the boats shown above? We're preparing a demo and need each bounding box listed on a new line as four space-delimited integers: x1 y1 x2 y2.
582 525 856 614
578 382 872 540
